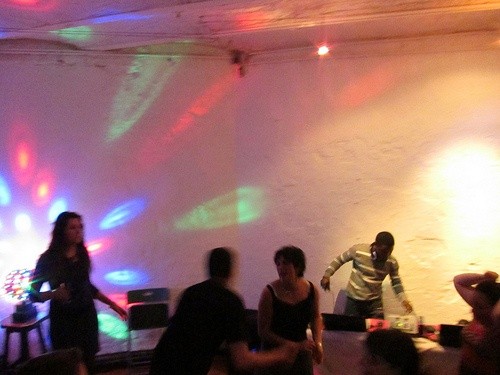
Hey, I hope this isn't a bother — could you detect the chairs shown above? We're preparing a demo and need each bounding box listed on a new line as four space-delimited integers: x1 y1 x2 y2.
126 287 171 366
321 313 367 332
439 324 467 348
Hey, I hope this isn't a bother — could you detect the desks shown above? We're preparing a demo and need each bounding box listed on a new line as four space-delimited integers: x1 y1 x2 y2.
0 312 50 364
306 329 465 375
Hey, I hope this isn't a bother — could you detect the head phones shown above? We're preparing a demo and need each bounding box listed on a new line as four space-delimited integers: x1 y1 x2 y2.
370 242 377 261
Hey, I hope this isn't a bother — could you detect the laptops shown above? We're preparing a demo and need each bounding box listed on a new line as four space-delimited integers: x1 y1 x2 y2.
440 324 466 348
320 313 366 332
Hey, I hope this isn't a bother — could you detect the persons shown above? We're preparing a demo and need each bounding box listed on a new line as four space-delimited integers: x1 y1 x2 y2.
321 232 413 318
453 271 500 375
258 245 323 375
150 248 298 375
28 212 127 364
364 328 419 375
